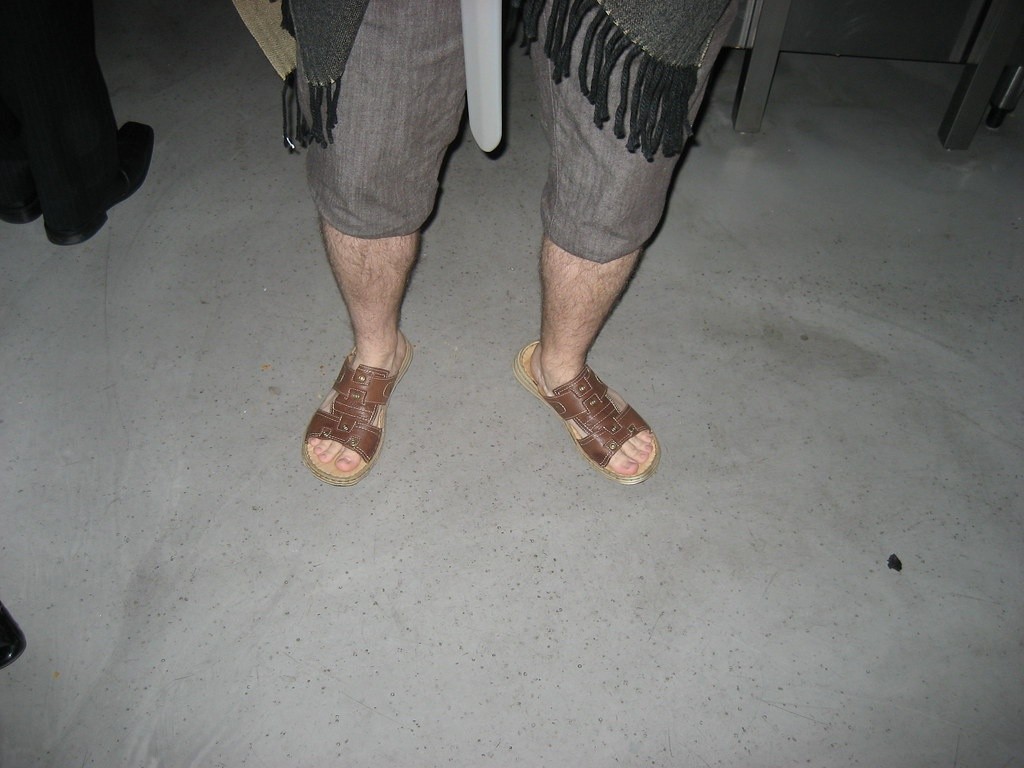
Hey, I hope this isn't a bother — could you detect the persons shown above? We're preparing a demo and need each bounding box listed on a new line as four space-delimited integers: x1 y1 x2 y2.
0 0 155 247
280 0 741 486
0 600 27 669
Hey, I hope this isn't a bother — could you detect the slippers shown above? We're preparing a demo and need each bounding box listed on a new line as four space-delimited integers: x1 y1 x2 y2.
302 329 413 487
510 341 661 487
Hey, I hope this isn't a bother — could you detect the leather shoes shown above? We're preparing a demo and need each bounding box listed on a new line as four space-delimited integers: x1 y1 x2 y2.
4 119 155 246
0 601 26 668
